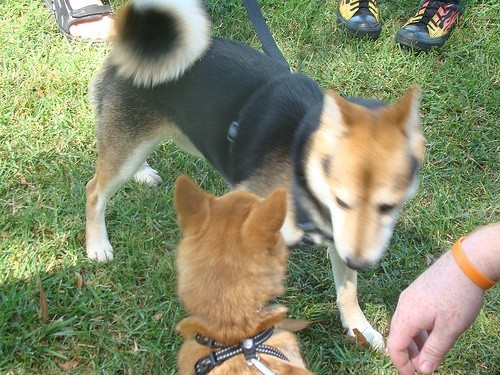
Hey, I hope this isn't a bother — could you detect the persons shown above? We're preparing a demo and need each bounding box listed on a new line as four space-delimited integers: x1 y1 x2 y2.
50 0 117 44
386 220 500 375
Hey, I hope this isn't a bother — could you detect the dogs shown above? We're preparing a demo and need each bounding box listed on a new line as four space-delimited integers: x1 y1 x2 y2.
86 0 427 356
173 175 315 375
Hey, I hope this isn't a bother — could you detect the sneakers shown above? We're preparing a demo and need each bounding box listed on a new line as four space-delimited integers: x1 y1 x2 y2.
395 0 465 54
337 0 383 40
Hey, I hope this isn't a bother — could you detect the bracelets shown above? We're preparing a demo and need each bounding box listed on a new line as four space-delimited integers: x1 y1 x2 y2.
449 234 498 290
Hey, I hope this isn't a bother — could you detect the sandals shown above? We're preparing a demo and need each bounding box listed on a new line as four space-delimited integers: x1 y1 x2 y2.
46 0 116 45
